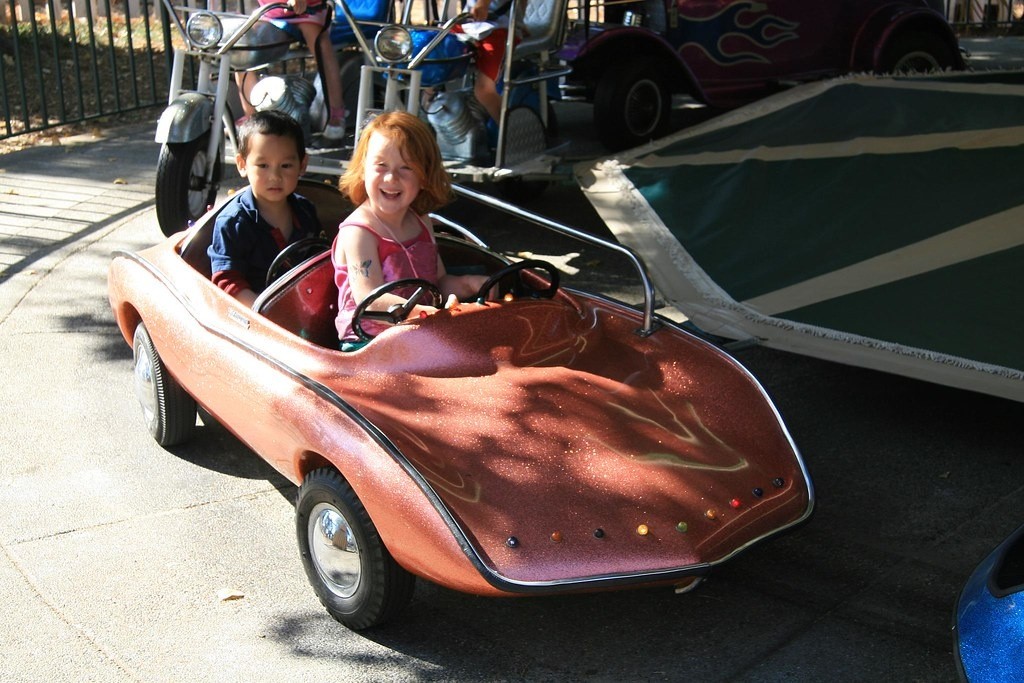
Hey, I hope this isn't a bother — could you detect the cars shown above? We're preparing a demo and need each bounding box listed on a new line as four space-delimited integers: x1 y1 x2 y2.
106 177 821 632
561 0 964 150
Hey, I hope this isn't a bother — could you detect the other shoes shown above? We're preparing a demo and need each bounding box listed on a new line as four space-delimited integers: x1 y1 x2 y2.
323 108 350 139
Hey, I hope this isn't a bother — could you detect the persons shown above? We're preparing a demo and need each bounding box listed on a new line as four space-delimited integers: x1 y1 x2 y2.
223 0 528 155
207 110 499 352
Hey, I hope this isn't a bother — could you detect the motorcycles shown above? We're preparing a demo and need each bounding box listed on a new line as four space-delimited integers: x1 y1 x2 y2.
154 0 574 236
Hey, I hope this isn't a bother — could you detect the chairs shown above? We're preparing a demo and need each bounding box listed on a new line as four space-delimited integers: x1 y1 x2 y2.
464 0 572 78
259 244 471 346
324 0 396 43
180 174 354 280
